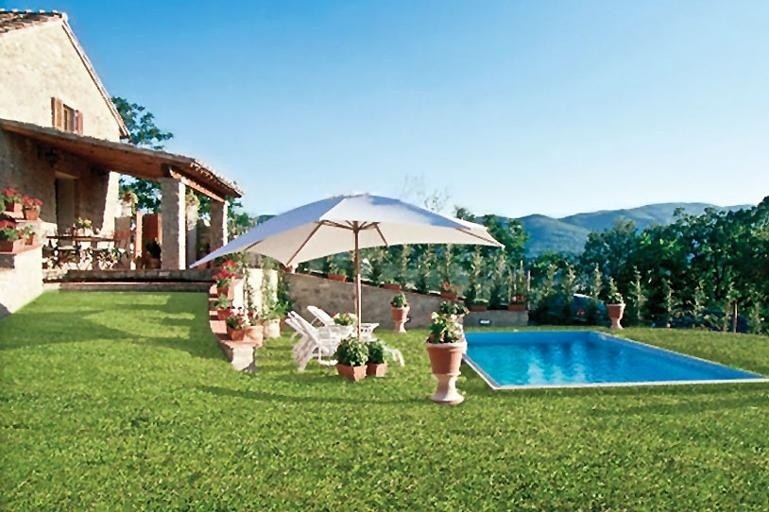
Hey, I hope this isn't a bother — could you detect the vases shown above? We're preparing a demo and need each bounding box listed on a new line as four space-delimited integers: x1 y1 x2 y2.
72 227 94 237
0 234 39 252
225 325 264 349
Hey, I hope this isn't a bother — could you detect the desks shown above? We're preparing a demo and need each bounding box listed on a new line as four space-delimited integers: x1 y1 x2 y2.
47 235 120 271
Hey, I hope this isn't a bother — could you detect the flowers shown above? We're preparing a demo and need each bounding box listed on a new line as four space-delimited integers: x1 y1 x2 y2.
0 188 43 241
212 260 260 329
73 216 93 229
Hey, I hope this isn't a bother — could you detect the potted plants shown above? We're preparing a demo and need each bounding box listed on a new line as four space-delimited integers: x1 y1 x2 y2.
426 312 467 405
607 293 626 330
439 302 469 324
259 304 281 339
364 340 388 378
391 295 410 332
333 337 369 381
328 263 347 282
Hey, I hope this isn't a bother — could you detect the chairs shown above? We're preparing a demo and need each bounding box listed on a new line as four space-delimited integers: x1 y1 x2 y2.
285 305 379 370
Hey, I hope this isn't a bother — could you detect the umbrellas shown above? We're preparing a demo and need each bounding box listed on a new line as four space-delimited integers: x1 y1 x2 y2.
187 192 506 340
